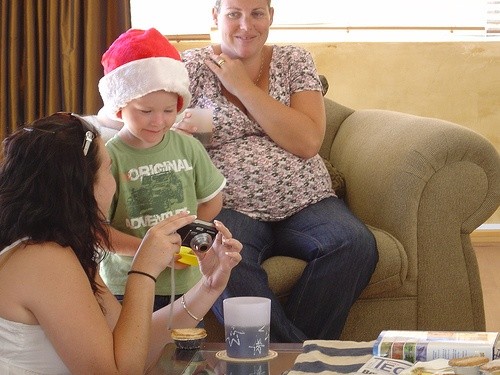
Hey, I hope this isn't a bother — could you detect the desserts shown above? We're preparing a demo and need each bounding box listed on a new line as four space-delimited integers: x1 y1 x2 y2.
171 328 207 349
448 356 500 375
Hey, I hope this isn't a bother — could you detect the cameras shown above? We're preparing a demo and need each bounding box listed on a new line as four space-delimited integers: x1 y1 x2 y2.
175 218 219 252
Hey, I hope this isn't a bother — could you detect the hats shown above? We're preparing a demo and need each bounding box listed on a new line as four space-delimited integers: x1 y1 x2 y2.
97 28 191 121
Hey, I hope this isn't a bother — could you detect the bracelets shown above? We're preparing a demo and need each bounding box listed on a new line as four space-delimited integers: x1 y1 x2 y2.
181 295 203 321
128 270 157 283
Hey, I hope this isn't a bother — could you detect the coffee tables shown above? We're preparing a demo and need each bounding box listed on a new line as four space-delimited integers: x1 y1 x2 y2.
152 339 500 375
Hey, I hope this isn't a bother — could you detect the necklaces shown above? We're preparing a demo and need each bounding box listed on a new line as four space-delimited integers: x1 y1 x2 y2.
255 49 263 87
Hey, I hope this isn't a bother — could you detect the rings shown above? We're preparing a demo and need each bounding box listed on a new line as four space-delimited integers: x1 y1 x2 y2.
219 59 225 65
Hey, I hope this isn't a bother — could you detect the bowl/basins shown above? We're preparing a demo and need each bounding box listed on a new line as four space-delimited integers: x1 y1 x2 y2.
170 329 208 350
453 365 490 375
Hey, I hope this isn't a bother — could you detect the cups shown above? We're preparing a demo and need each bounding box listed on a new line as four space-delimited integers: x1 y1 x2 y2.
223 296 272 358
226 360 269 375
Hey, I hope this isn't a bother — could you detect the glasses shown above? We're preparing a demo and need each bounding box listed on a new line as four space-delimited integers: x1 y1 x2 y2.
49 111 98 156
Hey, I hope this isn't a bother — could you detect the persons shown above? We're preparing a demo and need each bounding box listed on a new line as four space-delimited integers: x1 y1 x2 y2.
0 113 242 375
179 0 379 344
98 29 227 331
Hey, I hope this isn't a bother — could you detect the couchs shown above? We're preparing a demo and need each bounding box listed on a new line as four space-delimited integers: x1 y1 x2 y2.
206 97 500 342
